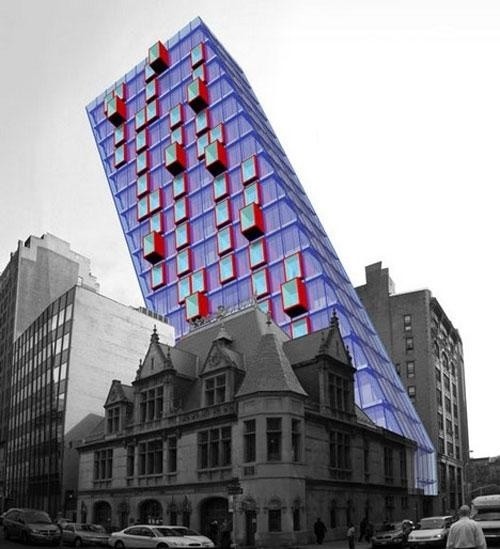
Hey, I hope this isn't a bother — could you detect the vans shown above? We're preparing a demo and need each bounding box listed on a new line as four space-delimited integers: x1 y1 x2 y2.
467 494 500 545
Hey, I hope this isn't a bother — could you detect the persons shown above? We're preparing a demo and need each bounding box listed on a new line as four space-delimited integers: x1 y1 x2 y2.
314 517 328 544
219 519 233 549
208 519 219 545
446 505 487 549
358 517 390 544
345 522 356 549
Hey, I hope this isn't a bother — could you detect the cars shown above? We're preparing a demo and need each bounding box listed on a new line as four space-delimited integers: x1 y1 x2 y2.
58 518 215 549
0 507 62 544
371 519 415 548
407 516 457 549
